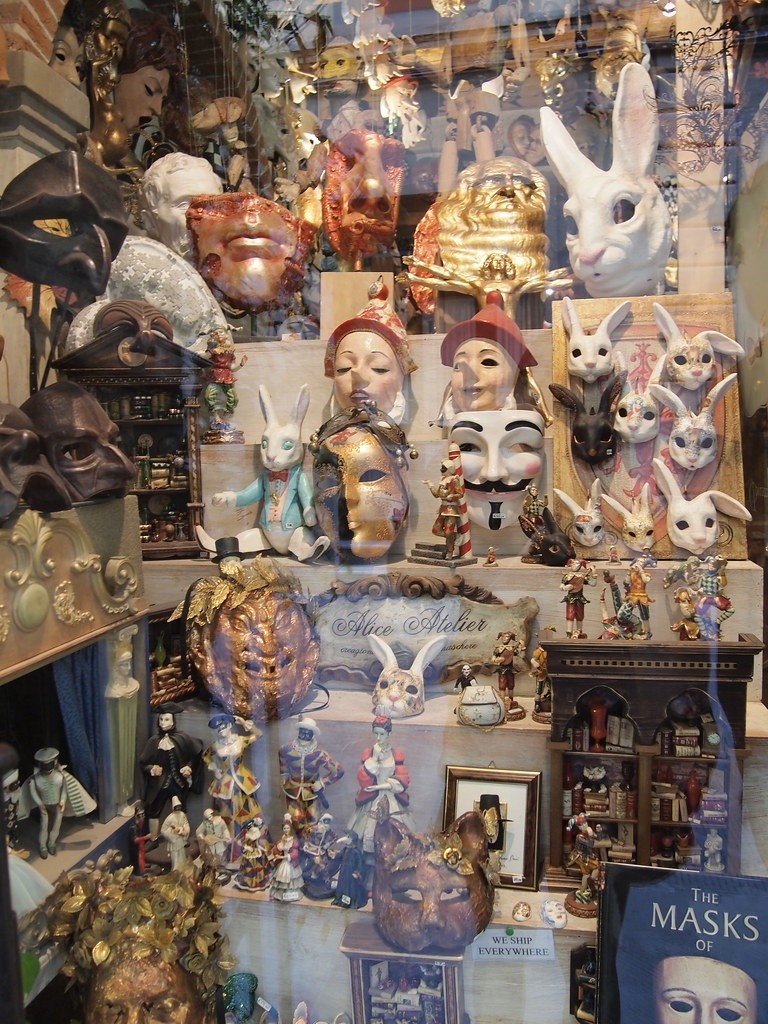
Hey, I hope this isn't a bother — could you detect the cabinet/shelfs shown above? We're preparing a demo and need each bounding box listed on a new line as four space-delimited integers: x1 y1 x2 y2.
51 319 216 559
534 631 765 894
339 923 468 1024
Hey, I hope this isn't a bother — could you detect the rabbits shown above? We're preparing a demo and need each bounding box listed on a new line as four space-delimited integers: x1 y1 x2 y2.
195 383 331 562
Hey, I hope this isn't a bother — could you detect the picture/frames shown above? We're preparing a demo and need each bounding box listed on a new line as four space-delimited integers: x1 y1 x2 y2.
441 766 542 893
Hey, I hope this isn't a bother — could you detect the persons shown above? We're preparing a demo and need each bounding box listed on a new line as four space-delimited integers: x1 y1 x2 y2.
1 1 767 1024
